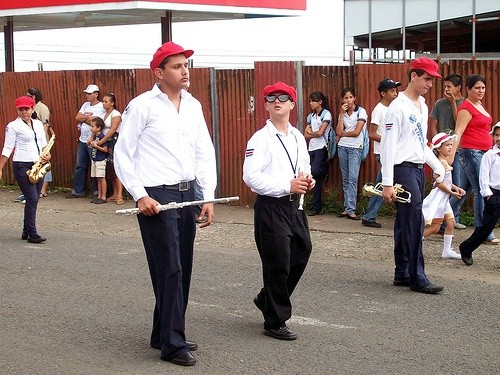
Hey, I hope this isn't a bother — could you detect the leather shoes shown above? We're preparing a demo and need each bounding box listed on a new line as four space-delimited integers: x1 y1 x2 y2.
186 340 198 349
96 198 108 204
442 251 461 260
394 280 409 287
337 213 347 217
91 198 99 203
22 234 28 240
253 294 266 320
27 236 47 243
263 326 298 340
308 210 320 216
362 218 381 228
66 193 78 198
169 351 196 366
416 282 444 293
346 214 361 220
459 244 473 266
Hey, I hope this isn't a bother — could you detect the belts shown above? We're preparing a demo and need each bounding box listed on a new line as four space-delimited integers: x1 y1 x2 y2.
394 161 424 168
281 193 297 201
155 180 193 191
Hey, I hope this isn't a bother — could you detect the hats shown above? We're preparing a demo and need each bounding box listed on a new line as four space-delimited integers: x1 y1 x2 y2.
431 133 454 151
489 121 500 139
15 96 36 109
83 84 99 93
410 57 442 79
377 79 401 92
262 82 296 103
150 42 194 73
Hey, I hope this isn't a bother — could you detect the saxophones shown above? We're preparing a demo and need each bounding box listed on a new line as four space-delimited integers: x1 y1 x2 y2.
26 120 56 183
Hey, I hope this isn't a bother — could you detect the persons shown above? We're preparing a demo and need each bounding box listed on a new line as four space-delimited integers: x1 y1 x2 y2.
335 86 368 220
112 41 218 366
0 96 52 243
26 88 52 197
241 81 316 340
459 120 500 266
437 74 500 245
304 91 332 216
429 74 466 229
65 84 107 198
422 132 466 260
87 116 108 204
87 92 124 205
362 78 401 228
380 57 445 293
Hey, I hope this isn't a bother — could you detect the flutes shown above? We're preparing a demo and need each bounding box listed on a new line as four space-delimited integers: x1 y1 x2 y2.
298 170 307 210
115 196 240 217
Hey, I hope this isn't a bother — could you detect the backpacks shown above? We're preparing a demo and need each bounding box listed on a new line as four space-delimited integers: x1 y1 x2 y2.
357 108 369 160
308 110 336 161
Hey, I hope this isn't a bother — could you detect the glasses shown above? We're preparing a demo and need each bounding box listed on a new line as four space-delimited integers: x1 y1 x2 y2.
264 94 292 102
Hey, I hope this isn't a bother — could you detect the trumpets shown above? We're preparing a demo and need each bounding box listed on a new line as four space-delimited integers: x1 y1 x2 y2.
362 182 411 203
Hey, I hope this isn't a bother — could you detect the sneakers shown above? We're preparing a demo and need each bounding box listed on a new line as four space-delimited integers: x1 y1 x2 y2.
14 194 25 201
454 223 466 228
485 238 500 245
194 207 208 223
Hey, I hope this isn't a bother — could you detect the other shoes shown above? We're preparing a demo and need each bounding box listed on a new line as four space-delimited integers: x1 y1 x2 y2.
117 198 124 204
107 196 116 201
39 192 48 197
